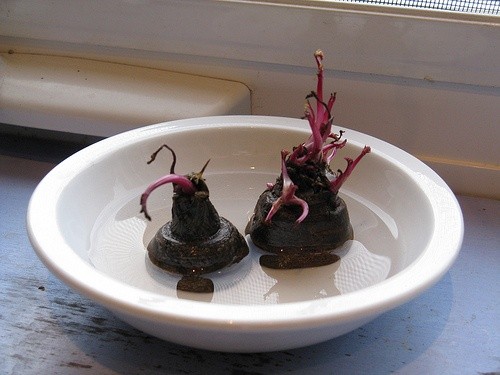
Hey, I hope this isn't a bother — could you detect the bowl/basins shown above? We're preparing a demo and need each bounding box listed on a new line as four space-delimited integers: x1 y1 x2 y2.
24 116 464 352
